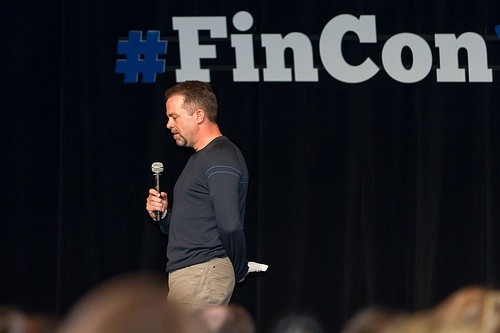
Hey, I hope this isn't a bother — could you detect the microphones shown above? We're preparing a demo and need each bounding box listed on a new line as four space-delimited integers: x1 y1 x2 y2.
151 162 164 221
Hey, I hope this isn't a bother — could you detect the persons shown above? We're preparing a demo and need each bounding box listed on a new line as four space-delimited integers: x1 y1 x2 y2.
146 81 249 306
